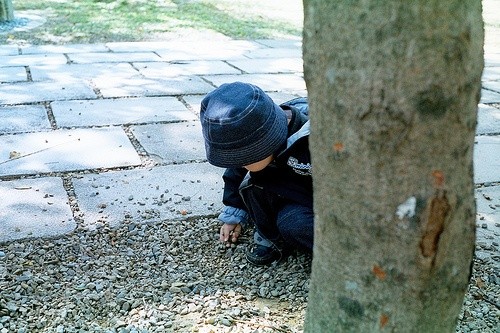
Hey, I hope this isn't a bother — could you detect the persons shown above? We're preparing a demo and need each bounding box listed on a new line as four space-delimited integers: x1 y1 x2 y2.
199 80 314 266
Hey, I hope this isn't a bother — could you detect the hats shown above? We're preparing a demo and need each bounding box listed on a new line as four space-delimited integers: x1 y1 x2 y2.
200 81 288 168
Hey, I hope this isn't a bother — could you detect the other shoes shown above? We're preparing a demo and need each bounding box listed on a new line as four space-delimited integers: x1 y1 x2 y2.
246 243 298 266
